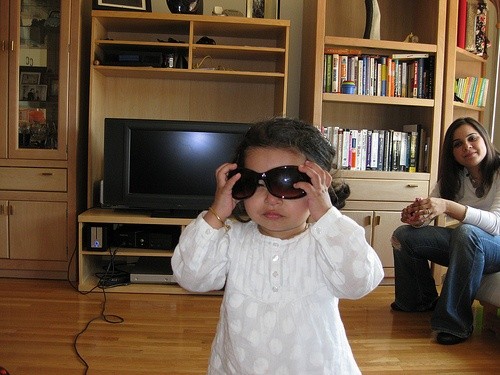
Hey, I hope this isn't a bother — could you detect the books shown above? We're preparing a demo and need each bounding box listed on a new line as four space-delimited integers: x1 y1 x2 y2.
321 46 490 173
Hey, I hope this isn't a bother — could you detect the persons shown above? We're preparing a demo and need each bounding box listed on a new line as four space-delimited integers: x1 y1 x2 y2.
390 117 500 344
172 114 385 375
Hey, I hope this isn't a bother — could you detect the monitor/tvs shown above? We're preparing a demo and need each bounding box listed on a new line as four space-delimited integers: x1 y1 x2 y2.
99 118 254 220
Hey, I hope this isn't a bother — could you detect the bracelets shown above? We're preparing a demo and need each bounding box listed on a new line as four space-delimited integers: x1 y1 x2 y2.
208 206 224 225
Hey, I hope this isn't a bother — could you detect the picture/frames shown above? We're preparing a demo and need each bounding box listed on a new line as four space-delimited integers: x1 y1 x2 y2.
93 0 153 12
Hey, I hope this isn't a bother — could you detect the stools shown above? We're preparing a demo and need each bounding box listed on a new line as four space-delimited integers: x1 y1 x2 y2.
441 274 500 337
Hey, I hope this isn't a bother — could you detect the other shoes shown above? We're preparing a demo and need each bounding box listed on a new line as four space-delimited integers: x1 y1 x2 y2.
390 300 437 311
436 330 464 345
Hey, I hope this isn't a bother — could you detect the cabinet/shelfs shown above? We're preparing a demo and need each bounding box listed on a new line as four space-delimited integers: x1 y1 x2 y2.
86 10 292 207
306 0 446 284
0 0 83 280
75 208 255 295
442 0 491 157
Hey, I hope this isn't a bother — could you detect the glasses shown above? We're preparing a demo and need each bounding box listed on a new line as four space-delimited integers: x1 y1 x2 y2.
227 165 314 200
